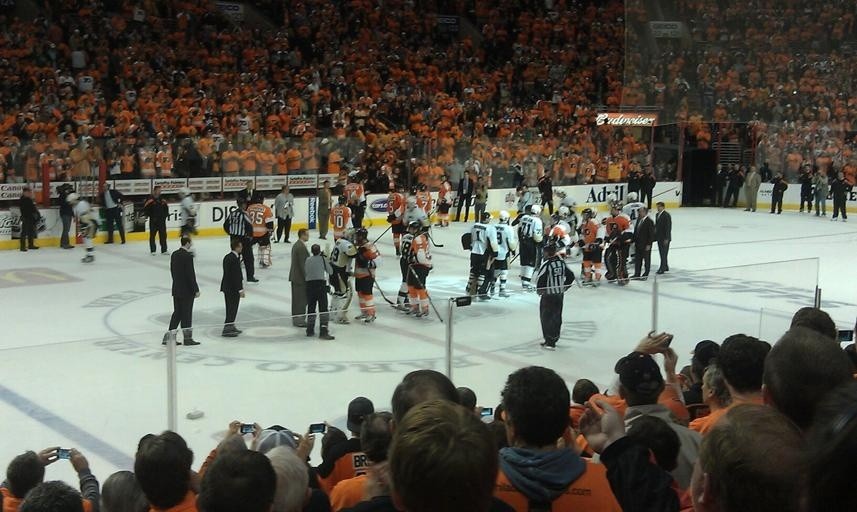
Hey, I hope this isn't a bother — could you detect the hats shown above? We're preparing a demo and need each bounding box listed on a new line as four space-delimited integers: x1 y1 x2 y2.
614 350 661 392
695 341 719 366
347 396 375 434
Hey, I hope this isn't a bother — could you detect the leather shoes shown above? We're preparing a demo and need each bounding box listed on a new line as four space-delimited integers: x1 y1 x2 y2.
184 340 201 346
222 323 243 336
452 217 468 222
630 266 673 280
274 237 292 243
20 240 127 252
162 338 182 346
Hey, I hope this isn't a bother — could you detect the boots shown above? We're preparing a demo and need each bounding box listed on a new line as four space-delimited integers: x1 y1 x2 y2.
435 219 451 228
578 275 630 286
246 275 261 285
541 338 557 350
462 283 539 301
330 312 376 323
398 302 431 319
307 326 334 339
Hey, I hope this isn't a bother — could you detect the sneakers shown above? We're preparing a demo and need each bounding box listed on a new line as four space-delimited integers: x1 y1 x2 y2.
151 250 172 255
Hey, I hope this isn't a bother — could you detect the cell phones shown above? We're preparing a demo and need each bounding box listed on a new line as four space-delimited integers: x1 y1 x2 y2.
838 330 853 341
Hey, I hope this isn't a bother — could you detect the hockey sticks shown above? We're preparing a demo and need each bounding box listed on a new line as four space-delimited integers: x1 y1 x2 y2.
428 234 444 248
374 279 395 304
364 210 372 230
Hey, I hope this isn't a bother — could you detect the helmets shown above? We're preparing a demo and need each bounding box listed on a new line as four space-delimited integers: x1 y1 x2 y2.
339 195 345 204
389 174 447 195
344 227 368 243
407 221 423 234
178 187 192 198
480 191 646 223
250 192 266 204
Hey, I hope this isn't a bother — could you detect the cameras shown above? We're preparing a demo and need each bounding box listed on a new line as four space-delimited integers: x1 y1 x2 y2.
57 448 70 459
480 408 492 416
660 334 673 353
240 424 254 433
310 424 325 433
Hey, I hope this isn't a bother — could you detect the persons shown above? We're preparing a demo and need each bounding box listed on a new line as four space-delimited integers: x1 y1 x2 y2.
2 310 857 512
288 229 310 328
1 2 856 269
161 236 200 345
302 243 334 340
351 227 379 324
534 242 574 350
462 212 492 303
487 210 516 298
223 194 260 283
405 221 432 317
627 207 654 281
394 219 417 312
325 227 356 324
513 202 634 295
654 201 672 275
218 240 246 338
